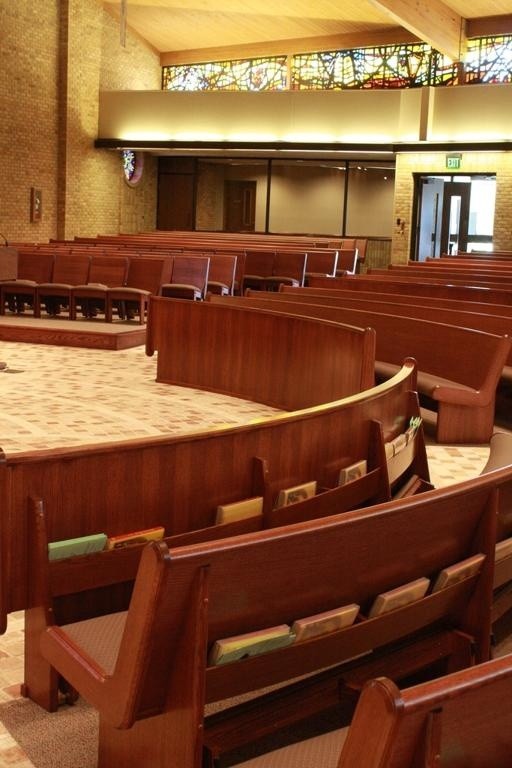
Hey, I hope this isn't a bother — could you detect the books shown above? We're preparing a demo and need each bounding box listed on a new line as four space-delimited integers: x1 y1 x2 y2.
494 536 511 561
277 481 317 508
291 604 360 640
108 526 165 551
217 497 264 526
339 460 368 483
432 554 486 591
48 532 107 562
204 624 296 667
367 577 430 619
386 415 423 460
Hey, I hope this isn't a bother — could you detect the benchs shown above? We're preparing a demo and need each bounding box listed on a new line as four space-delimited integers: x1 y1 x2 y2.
146 250 512 444
21 433 512 767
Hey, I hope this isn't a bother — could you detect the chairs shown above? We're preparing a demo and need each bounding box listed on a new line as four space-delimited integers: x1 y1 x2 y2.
0 231 359 325
